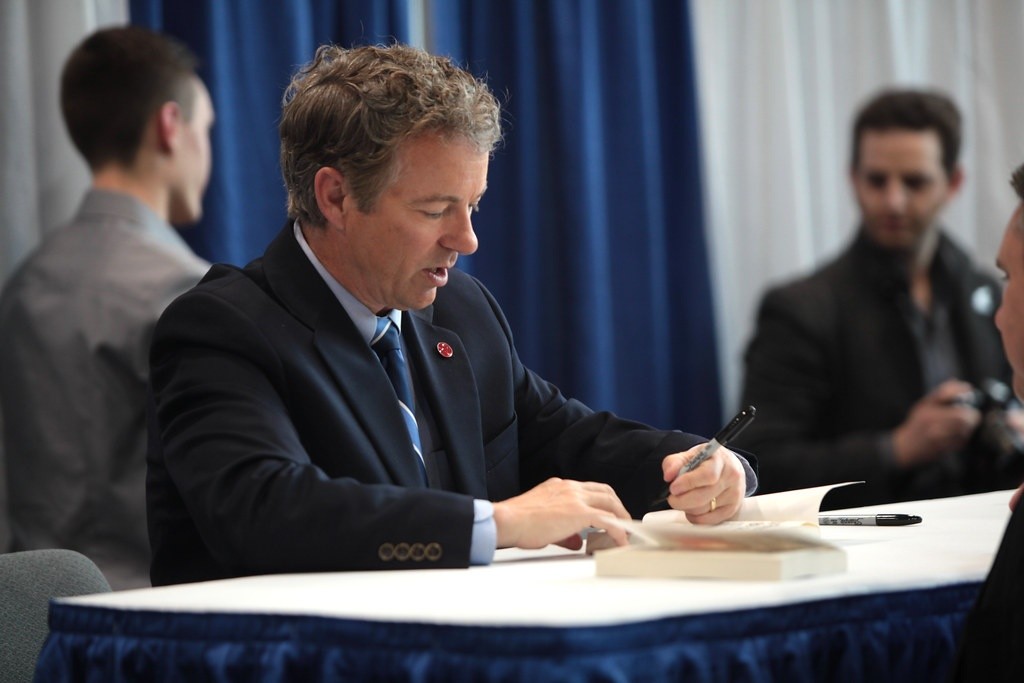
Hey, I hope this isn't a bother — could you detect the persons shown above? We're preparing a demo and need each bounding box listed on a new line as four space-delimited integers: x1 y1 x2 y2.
955 162 1024 683
0 24 216 592
737 85 1023 512
145 44 758 588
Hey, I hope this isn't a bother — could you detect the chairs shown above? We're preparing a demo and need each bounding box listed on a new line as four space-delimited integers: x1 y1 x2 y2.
1 548 114 682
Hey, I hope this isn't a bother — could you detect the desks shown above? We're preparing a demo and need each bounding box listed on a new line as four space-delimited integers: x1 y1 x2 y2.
31 481 1024 683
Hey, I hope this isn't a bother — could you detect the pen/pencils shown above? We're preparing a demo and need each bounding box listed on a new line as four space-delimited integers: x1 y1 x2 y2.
653 406 755 506
806 514 921 527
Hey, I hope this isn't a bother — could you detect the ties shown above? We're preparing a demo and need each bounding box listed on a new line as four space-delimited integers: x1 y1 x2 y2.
370 317 430 490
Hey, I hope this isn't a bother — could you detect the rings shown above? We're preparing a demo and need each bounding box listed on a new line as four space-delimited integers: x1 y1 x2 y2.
710 497 716 512
